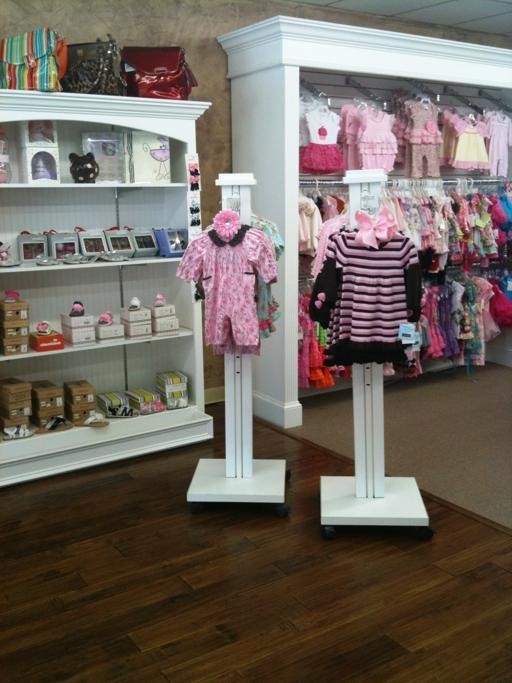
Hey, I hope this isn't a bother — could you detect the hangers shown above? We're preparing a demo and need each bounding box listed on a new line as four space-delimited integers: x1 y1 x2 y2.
380 177 500 206
299 178 347 201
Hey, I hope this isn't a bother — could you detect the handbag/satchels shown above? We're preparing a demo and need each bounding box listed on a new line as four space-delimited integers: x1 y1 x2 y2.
59 33 128 96
0 27 70 94
121 44 200 103
